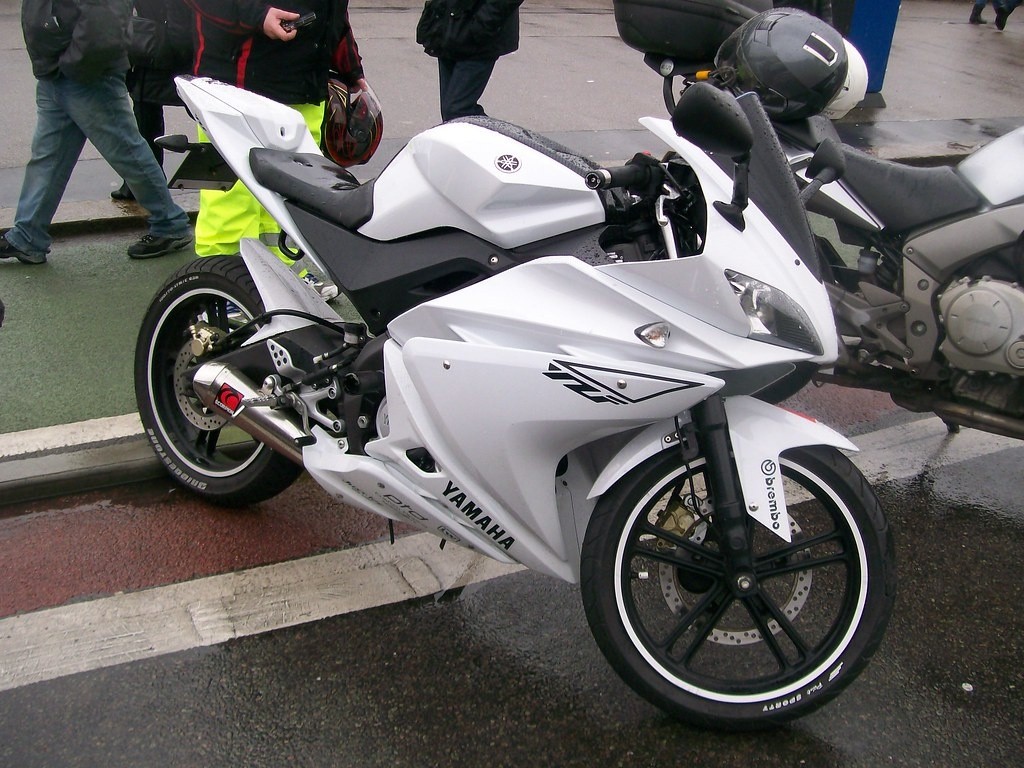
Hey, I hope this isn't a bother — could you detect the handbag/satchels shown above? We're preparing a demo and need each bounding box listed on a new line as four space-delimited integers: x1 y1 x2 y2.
128 16 168 62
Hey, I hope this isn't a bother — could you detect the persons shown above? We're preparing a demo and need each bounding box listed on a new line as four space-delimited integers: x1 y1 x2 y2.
416 0 524 122
0 0 367 324
968 0 1021 30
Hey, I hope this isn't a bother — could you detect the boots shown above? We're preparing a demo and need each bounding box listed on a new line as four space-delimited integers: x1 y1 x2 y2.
970 3 987 24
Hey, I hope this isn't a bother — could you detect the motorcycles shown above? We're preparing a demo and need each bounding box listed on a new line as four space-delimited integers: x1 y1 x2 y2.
132 74 900 735
614 0 1023 439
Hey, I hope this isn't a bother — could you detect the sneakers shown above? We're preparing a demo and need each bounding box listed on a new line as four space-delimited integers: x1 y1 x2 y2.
0 236 47 264
127 231 194 258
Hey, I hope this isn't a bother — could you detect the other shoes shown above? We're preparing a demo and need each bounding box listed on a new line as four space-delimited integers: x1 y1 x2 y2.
995 6 1008 31
111 188 135 201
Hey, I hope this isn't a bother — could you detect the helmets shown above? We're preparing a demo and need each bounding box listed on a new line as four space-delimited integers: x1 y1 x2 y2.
819 36 868 120
713 7 849 122
320 68 383 168
